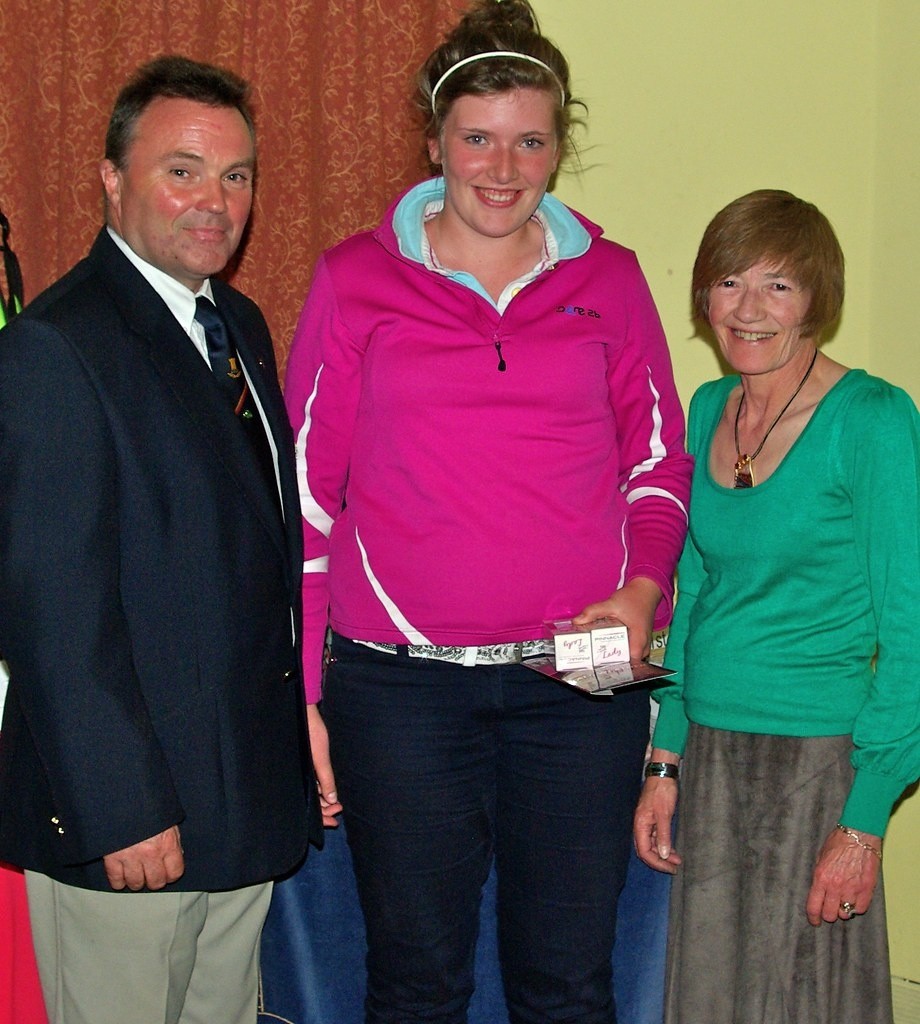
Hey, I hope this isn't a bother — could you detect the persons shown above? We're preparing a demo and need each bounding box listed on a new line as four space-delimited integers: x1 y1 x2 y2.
0 53 323 1023
282 0 694 1023
630 189 920 1024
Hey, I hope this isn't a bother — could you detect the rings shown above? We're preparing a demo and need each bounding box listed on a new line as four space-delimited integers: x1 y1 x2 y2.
839 902 855 913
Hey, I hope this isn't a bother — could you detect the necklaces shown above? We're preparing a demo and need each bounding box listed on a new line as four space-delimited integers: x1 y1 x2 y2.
734 348 818 490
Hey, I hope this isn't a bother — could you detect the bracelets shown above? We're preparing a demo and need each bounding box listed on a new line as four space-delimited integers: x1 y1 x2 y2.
836 823 883 860
644 762 680 780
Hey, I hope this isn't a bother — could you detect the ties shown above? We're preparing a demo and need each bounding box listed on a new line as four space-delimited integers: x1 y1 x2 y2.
194 295 289 555
